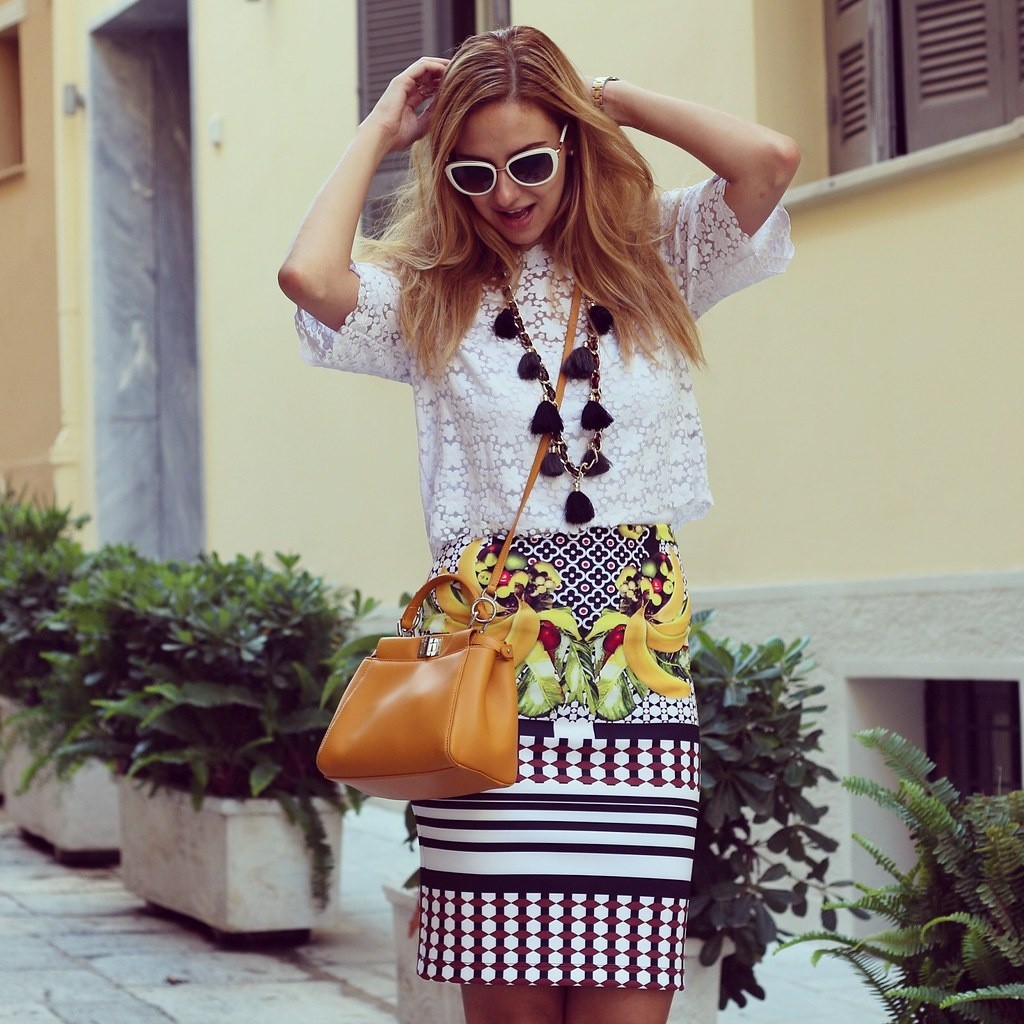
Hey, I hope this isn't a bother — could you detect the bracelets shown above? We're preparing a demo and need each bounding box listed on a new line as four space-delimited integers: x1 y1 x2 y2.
591 76 618 118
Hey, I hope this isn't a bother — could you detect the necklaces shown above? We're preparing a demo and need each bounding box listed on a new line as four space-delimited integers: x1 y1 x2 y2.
493 255 615 524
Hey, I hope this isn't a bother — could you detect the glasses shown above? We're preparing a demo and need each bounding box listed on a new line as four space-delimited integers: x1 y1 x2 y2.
445 122 570 197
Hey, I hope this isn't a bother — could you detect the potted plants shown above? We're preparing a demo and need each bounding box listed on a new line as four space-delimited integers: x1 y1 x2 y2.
0 478 869 1024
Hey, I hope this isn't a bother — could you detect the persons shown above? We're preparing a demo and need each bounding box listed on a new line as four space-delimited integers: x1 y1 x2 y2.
278 25 802 1024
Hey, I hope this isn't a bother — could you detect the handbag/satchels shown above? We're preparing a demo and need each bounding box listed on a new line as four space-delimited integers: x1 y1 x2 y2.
315 574 520 799
